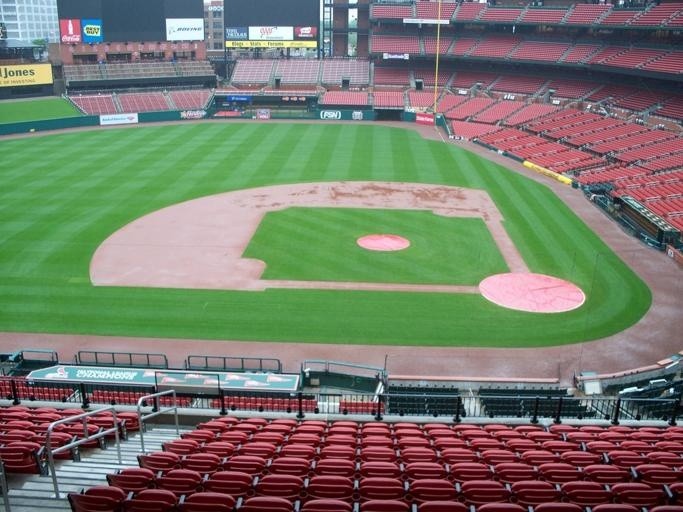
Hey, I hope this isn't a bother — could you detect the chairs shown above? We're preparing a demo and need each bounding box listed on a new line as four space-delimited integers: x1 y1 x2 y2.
1 2 373 127
372 0 683 251
0 347 683 512
